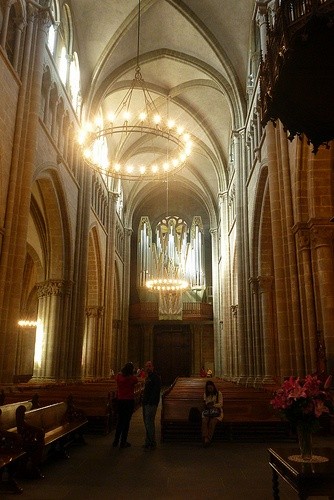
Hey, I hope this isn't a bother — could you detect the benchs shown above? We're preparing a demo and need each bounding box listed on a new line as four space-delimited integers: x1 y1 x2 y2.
160 376 296 444
0 377 145 493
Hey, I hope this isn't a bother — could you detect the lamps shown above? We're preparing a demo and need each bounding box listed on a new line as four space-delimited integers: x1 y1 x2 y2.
75 0 193 180
136 94 205 320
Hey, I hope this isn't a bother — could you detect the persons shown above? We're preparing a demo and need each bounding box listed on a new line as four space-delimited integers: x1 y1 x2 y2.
138 363 161 451
200 367 212 377
201 380 224 445
112 364 137 448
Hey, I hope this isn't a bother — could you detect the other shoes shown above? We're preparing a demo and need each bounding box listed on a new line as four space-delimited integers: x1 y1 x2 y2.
113 442 118 446
120 442 131 448
204 442 212 448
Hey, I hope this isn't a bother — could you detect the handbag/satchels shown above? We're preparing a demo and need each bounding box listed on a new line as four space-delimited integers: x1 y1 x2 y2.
204 406 220 416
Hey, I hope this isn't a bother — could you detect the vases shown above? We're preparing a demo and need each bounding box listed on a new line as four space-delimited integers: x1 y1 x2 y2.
298 427 312 460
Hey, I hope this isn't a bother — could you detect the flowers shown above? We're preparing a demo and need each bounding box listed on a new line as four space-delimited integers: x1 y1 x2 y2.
270 373 334 454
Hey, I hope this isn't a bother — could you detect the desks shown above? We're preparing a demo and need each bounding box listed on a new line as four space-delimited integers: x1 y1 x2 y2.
264 447 334 500
